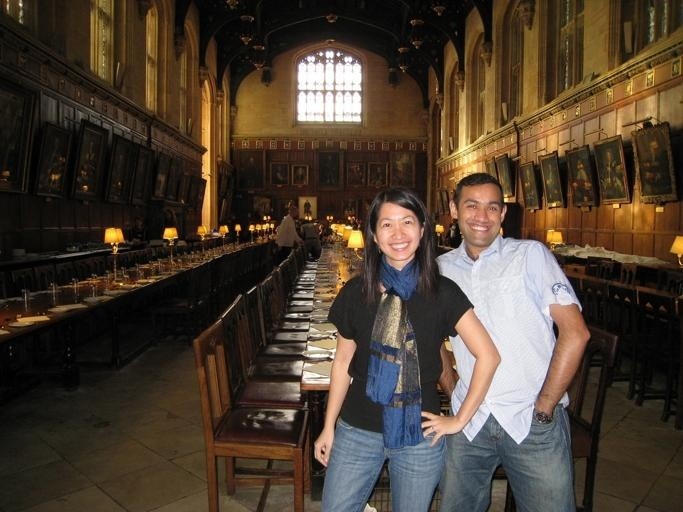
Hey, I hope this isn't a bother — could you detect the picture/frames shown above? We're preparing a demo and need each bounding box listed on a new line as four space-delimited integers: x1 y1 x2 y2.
237 148 415 192
341 198 375 218
434 190 450 215
33 118 206 210
485 153 514 198
298 196 317 219
519 122 679 209
0 76 38 195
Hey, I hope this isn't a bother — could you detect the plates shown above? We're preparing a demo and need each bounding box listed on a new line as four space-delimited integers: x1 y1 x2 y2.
8 241 252 327
301 248 334 360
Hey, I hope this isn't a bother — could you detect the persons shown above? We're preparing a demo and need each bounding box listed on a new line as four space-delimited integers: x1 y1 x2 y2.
312 185 503 511
523 136 673 207
127 207 323 267
302 198 312 216
431 172 590 512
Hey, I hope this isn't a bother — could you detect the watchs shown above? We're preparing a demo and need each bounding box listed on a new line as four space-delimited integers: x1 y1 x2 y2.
533 410 553 426
